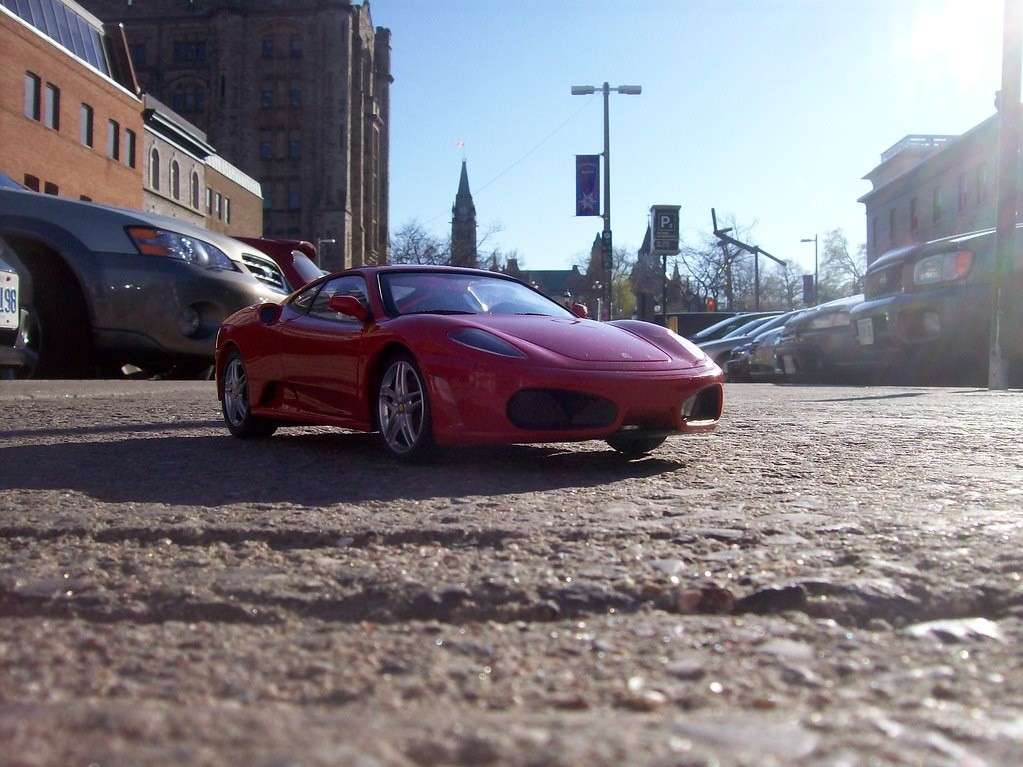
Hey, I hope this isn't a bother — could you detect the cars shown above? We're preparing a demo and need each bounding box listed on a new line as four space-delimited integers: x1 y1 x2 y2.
0 171 311 380
231 233 418 304
683 223 1023 387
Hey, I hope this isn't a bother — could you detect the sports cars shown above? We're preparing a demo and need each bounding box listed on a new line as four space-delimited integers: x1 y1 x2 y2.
212 261 726 470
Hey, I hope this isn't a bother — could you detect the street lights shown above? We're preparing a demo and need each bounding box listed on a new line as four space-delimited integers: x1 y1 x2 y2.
801 234 819 306
317 237 336 270
570 80 642 320
